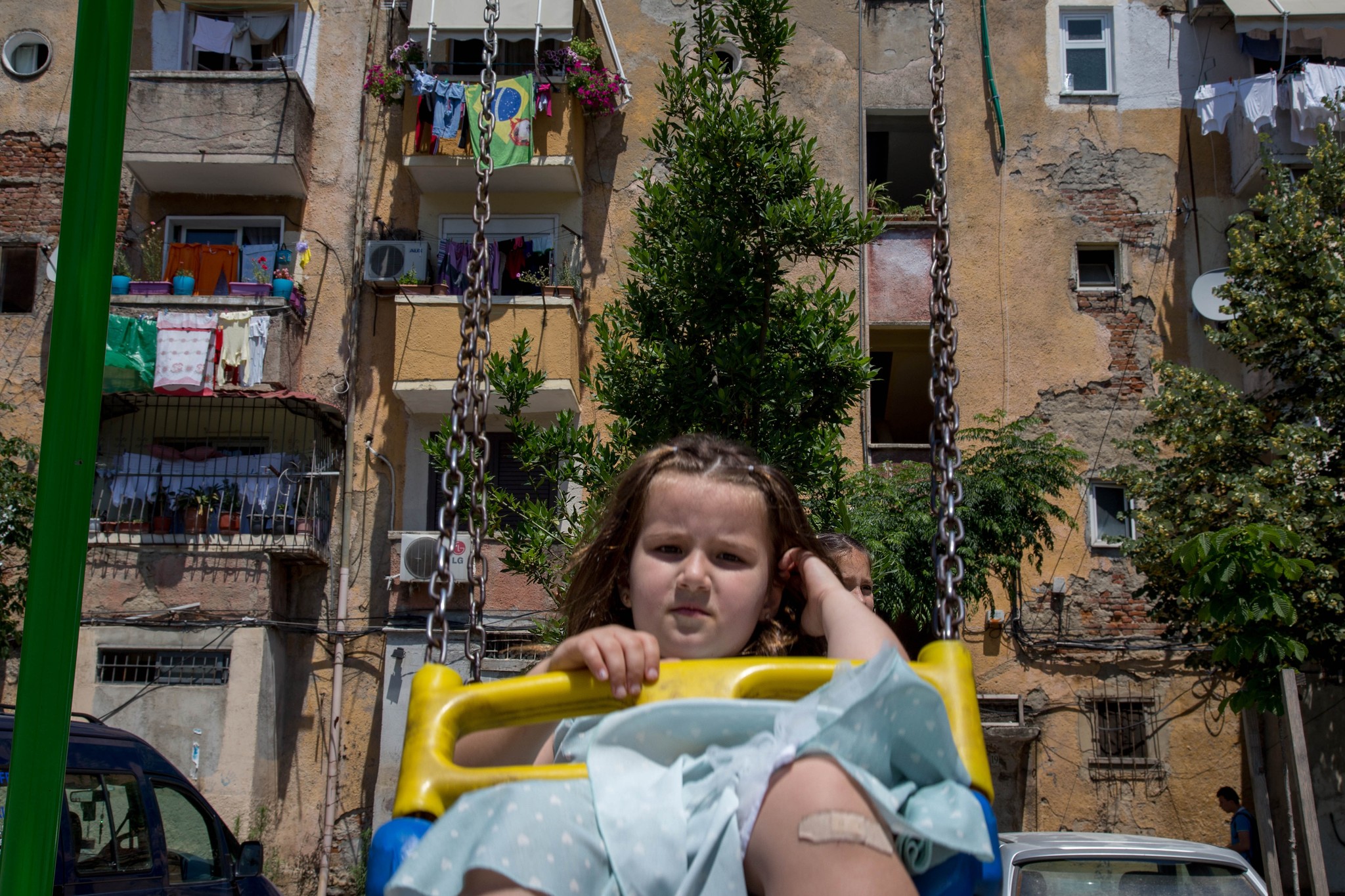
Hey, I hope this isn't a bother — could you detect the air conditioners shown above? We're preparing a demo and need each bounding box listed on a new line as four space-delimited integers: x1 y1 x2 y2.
363 240 431 287
400 534 475 583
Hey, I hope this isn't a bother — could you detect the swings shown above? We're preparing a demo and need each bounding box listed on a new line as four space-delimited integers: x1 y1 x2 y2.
368 0 1002 896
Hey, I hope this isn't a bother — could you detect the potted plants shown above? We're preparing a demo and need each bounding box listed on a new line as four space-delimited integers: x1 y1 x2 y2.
89 478 313 534
395 269 449 295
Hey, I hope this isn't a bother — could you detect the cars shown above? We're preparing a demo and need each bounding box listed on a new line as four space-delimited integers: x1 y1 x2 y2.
996 830 1272 895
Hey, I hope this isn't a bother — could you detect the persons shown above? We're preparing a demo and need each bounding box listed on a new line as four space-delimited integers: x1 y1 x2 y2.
384 432 994 896
1216 786 1263 876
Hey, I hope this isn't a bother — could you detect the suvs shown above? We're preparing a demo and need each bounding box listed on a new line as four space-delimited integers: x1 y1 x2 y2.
0 703 283 896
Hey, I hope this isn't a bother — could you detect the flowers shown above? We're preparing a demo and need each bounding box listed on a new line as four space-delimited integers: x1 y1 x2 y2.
539 36 627 114
241 255 269 283
174 262 193 276
273 268 291 279
517 247 578 297
363 41 425 103
114 234 131 277
136 221 167 280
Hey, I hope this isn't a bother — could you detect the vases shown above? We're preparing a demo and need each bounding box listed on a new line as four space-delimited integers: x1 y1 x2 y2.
173 276 195 295
129 281 173 295
543 286 574 300
273 279 293 298
576 55 590 67
389 84 404 99
228 282 272 297
111 276 131 295
